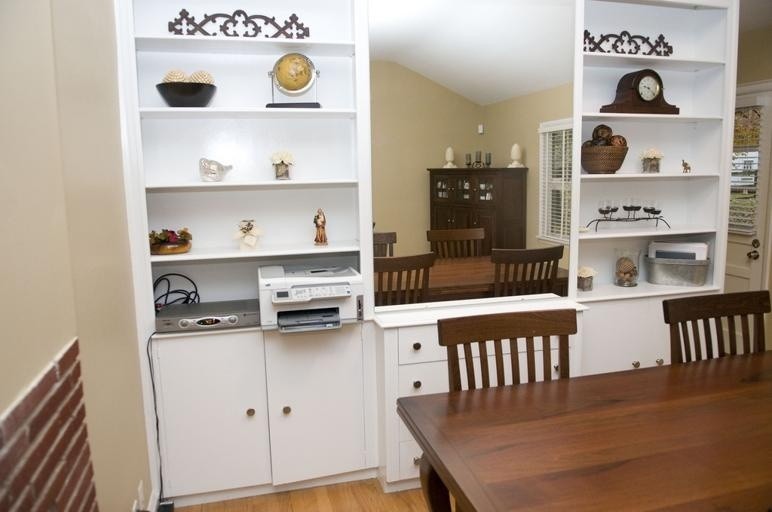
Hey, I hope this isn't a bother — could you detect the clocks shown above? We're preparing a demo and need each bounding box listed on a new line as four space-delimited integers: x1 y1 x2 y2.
598 68 683 115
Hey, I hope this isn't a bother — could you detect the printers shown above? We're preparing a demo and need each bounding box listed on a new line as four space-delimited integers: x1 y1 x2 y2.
257 264 362 334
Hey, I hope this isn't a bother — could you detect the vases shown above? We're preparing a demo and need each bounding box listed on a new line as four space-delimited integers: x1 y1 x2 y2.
149 243 192 257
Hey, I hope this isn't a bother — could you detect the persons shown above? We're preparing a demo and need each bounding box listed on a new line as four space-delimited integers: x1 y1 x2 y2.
313 208 329 246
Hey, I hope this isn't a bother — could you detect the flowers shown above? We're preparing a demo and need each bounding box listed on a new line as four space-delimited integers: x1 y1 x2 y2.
146 226 193 248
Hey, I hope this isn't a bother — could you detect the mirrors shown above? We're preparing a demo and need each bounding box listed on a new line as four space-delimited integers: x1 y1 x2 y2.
355 2 583 313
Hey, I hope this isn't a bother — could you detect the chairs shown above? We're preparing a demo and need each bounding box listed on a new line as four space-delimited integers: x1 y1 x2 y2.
373 228 568 304
436 308 578 392
662 291 772 364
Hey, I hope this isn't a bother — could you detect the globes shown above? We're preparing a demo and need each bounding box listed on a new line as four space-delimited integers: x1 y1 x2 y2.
266 52 320 108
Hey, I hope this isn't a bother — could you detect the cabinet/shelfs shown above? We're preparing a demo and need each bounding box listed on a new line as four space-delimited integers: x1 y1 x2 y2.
113 1 381 340
372 309 583 494
583 299 723 380
141 321 377 510
426 167 528 256
582 1 738 301
